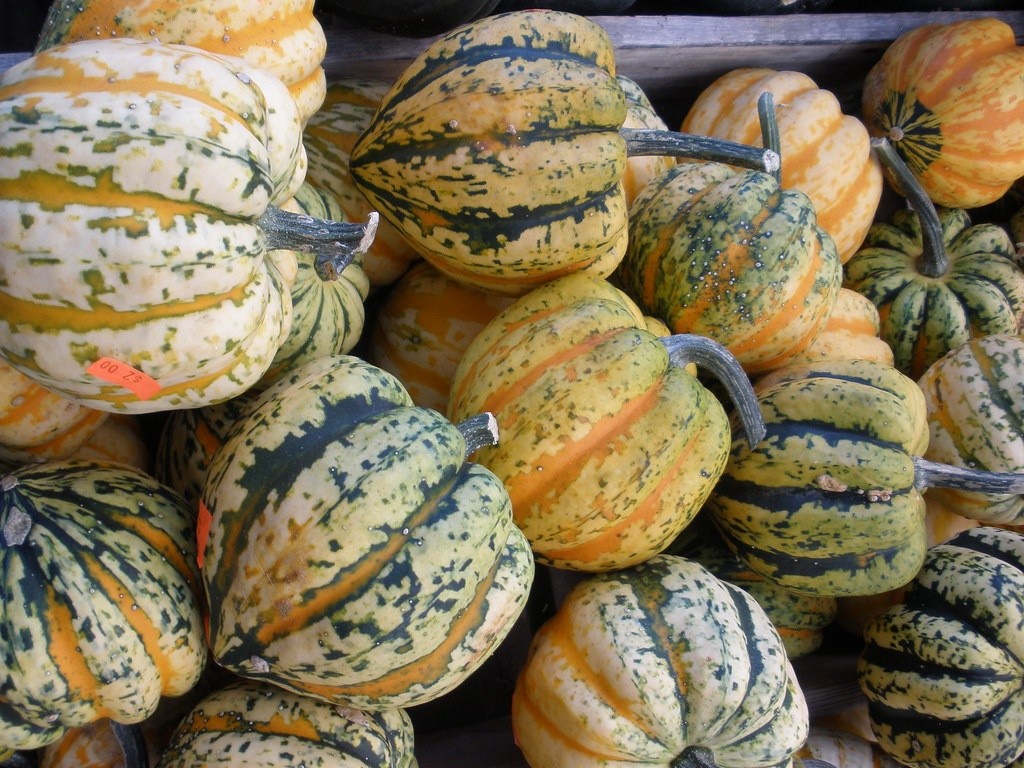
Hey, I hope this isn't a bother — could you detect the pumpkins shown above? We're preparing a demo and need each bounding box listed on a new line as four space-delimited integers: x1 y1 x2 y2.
0 0 1024 768
